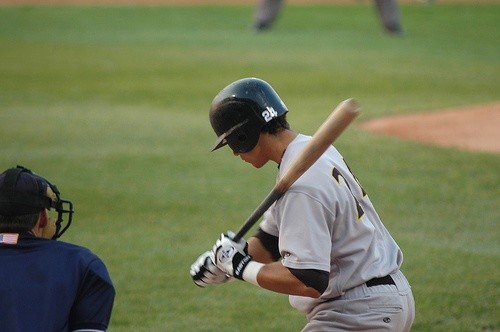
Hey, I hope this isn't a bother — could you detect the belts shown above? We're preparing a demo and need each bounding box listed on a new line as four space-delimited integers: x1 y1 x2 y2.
365 275 397 287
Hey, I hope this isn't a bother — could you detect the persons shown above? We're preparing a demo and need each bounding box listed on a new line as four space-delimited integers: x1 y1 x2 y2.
0 162 116 332
190 75 417 332
251 1 403 35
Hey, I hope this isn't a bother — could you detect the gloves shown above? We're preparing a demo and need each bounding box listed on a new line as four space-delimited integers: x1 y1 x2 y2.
189 251 232 289
209 229 265 288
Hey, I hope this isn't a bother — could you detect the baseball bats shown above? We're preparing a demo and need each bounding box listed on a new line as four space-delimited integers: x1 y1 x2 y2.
190 98 362 288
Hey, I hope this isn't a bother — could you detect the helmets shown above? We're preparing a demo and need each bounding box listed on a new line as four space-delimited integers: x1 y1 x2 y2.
208 77 288 152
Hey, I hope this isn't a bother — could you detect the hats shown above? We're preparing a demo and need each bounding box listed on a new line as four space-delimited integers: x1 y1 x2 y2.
0 164 51 216
